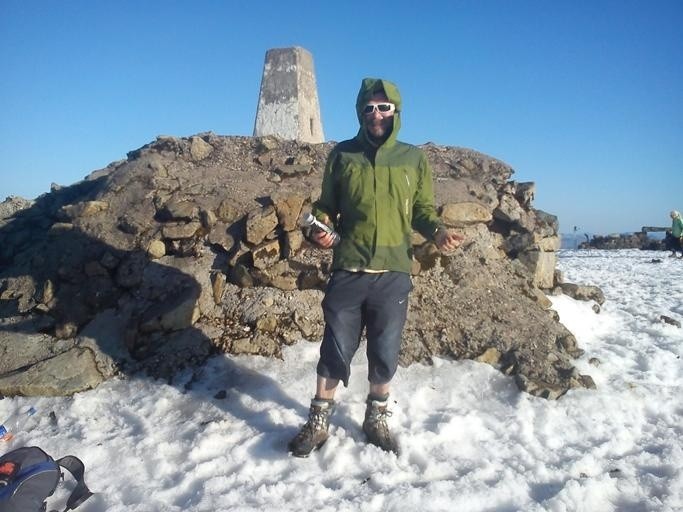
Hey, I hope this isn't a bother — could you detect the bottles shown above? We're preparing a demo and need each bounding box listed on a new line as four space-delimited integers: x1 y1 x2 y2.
304 213 340 246
0 407 37 443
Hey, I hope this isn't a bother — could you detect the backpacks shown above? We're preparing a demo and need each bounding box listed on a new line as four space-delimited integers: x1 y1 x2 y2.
0 446 93 512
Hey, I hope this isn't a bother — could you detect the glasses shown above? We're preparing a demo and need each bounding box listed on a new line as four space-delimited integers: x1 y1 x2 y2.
362 103 395 114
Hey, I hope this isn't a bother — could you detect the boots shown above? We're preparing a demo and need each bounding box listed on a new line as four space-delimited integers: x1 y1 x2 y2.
289 399 336 458
678 255 683 259
668 252 677 258
363 393 398 455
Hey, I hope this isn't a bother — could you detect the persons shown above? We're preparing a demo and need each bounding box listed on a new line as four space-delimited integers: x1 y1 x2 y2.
288 78 465 458
668 210 683 259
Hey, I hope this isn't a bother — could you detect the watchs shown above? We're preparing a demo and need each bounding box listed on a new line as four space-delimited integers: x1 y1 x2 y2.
429 225 449 235
